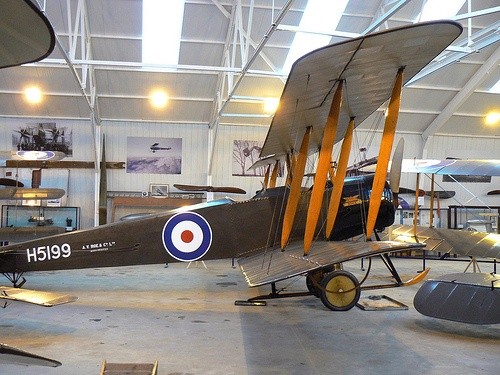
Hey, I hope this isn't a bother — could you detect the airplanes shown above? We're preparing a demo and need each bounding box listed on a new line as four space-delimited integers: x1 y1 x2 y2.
0 17 467 313
355 157 500 326
